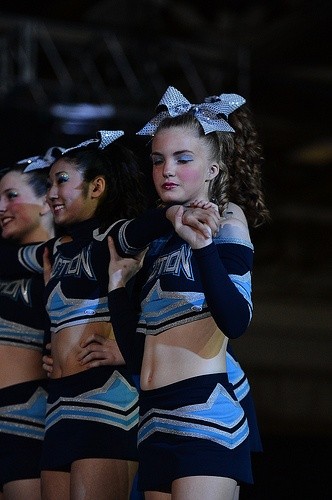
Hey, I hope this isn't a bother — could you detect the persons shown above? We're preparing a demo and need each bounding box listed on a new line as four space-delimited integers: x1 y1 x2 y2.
43 236 266 500
106 87 273 500
2 155 53 499
1 129 223 499
